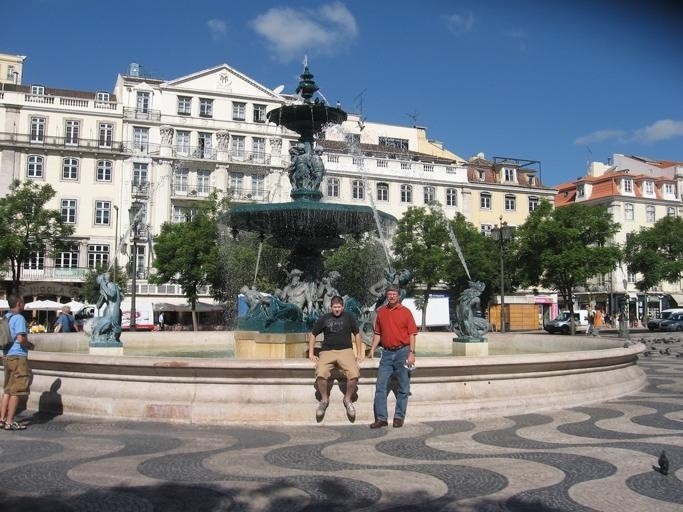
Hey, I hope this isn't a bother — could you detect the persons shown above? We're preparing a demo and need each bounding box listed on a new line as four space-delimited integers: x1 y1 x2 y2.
367 284 418 429
175 322 184 331
584 304 595 336
285 142 325 192
91 272 125 342
240 268 350 319
591 304 602 337
368 268 413 313
0 292 35 430
451 280 489 340
309 296 363 419
159 312 166 331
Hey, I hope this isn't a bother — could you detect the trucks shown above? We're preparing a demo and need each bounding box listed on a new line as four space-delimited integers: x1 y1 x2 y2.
545 309 597 335
74 305 153 331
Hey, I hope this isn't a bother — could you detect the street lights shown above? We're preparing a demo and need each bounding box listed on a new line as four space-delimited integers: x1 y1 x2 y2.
113 205 119 282
491 213 512 333
128 200 142 331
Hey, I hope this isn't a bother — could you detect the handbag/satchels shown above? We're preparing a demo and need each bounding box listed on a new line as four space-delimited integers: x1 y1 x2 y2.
53 322 63 333
69 322 76 332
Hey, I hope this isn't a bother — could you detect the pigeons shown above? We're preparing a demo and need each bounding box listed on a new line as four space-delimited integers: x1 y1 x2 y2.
658 449 669 476
623 337 683 357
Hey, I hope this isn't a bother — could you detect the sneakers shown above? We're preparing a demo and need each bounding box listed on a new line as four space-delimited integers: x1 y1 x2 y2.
0 419 27 430
315 400 328 417
343 399 355 418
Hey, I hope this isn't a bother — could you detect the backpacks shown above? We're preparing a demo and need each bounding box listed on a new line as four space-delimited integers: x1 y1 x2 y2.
0 313 19 350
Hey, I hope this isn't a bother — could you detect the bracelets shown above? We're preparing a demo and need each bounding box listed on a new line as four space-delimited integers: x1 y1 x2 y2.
409 350 416 354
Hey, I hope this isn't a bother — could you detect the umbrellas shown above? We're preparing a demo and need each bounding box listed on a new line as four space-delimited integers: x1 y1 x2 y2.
24 300 85 333
0 299 12 316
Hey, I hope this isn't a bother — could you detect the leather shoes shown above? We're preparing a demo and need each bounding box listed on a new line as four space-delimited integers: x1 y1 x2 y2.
384 344 409 351
393 418 403 427
370 419 388 429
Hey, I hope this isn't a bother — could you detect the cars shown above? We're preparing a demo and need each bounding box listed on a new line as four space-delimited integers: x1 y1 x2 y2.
647 308 683 331
